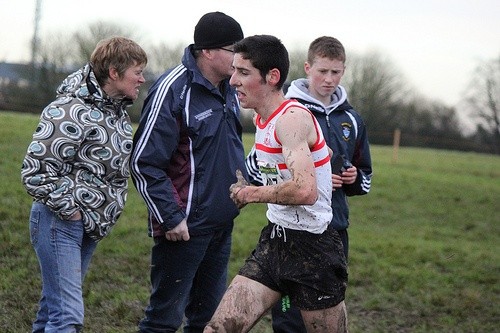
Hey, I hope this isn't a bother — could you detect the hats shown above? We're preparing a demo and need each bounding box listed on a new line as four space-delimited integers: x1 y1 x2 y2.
194 11 244 49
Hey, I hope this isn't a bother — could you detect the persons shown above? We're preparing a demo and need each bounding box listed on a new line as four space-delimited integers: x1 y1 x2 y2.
243 35 375 333
20 35 149 333
129 10 247 332
199 33 352 333
